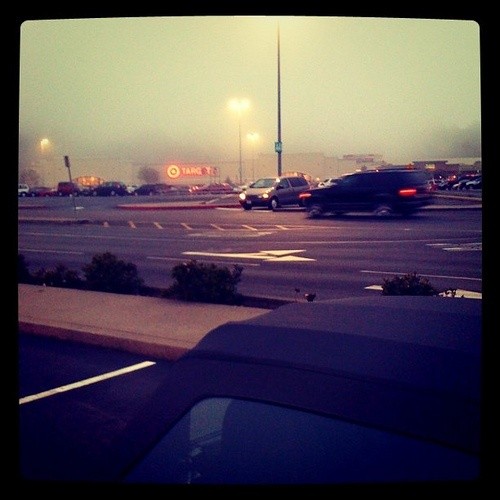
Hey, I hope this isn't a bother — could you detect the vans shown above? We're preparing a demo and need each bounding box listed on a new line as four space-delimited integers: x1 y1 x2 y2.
238 174 309 211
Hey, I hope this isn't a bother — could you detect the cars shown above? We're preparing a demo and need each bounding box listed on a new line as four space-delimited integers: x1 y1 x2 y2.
18 182 239 197
430 170 482 193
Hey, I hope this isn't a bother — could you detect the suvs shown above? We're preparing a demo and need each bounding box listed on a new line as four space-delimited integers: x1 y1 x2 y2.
298 168 434 220
